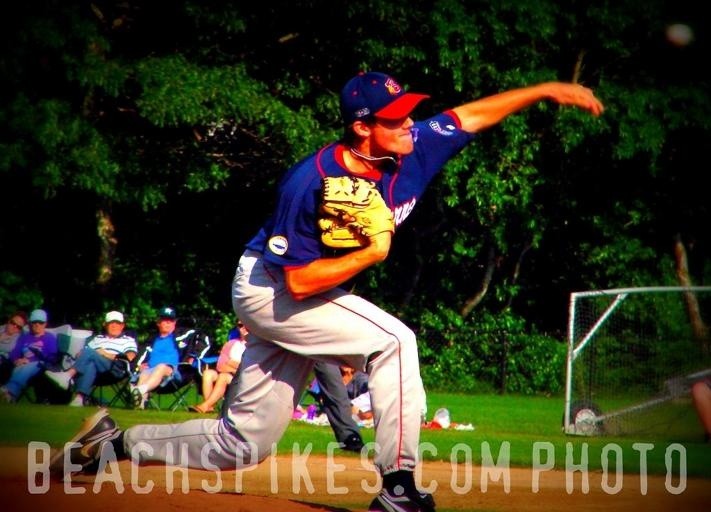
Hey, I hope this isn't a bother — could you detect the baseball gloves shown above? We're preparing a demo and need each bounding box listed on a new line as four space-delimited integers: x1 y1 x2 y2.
318 176 395 248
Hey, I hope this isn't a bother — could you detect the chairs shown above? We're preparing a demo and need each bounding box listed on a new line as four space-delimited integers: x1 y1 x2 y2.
34 325 240 412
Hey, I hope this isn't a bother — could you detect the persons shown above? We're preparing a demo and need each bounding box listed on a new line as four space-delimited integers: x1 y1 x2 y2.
44 309 140 406
187 317 253 414
334 360 375 426
127 307 211 407
692 379 711 437
44 69 608 512
0 308 62 406
0 311 26 381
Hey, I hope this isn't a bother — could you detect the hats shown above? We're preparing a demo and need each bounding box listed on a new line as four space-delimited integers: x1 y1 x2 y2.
158 307 176 319
338 70 431 127
28 309 47 323
104 311 124 323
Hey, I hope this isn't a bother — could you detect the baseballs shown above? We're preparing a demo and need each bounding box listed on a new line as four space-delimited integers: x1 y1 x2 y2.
667 24 691 46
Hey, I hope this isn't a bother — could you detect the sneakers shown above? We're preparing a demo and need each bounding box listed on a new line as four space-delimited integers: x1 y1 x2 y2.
45 370 70 390
36 406 123 486
131 385 143 407
368 487 436 511
187 405 203 414
69 397 83 407
340 435 374 454
